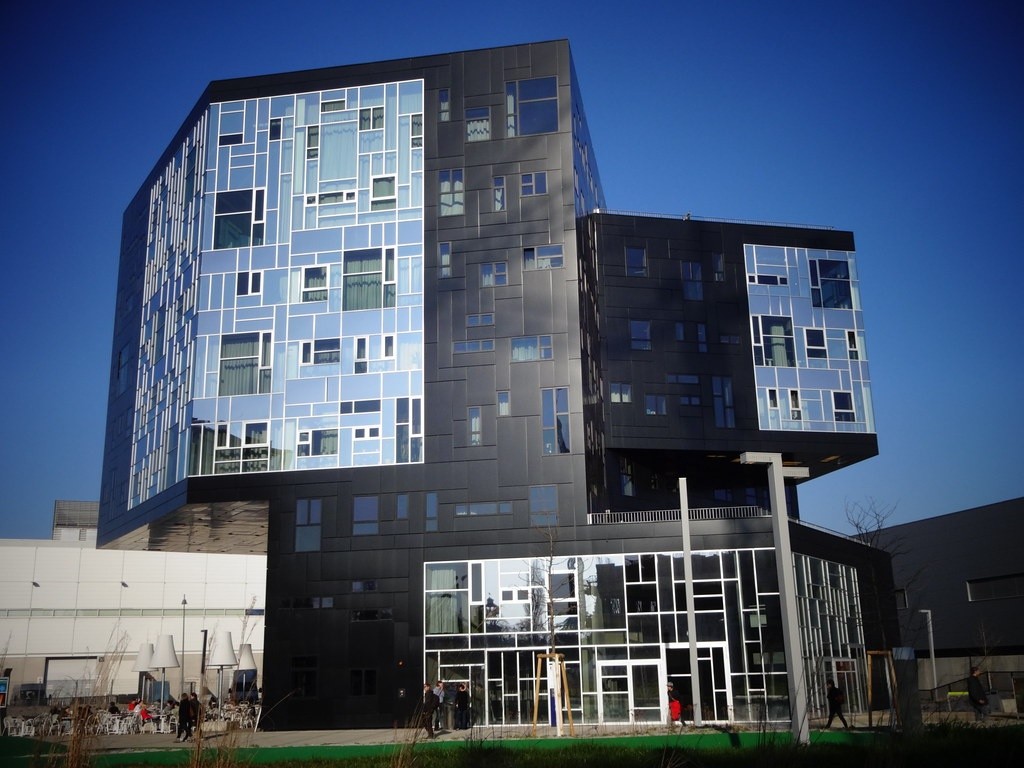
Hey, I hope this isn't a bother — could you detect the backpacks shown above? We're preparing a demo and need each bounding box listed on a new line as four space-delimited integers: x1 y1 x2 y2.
427 690 440 708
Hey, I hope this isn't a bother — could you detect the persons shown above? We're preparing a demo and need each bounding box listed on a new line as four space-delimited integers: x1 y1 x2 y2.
433 681 445 731
454 684 470 731
419 683 434 739
181 693 204 742
825 680 848 729
173 693 196 743
967 667 989 721
50 696 219 740
667 682 685 725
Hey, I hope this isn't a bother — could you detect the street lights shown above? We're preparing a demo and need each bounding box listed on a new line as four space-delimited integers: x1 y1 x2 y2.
179 594 188 702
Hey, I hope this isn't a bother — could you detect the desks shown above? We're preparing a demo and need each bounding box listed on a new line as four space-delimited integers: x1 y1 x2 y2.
61 717 76 736
152 715 168 733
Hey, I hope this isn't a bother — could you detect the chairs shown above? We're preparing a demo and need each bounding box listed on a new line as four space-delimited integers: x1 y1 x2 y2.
1 701 261 738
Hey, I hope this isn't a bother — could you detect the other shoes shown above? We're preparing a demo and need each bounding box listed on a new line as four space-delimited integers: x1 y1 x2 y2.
173 738 180 743
426 735 435 739
187 738 195 743
